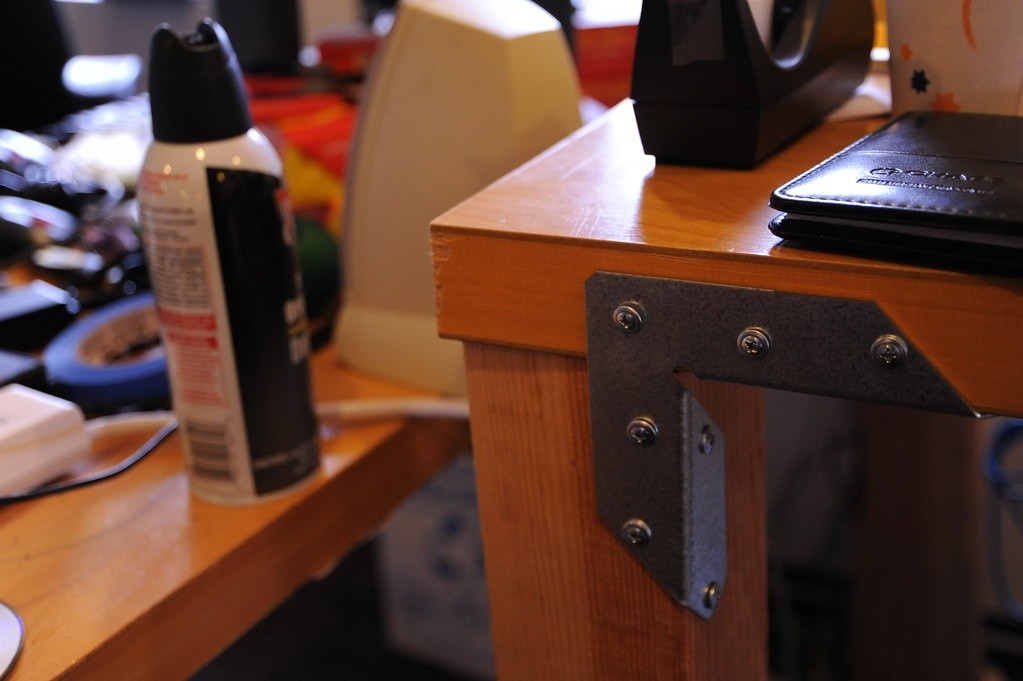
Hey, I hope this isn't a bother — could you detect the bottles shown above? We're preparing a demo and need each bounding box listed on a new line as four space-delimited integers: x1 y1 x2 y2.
136 16 325 502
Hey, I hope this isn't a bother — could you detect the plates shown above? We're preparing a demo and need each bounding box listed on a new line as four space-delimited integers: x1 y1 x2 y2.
0 599 27 680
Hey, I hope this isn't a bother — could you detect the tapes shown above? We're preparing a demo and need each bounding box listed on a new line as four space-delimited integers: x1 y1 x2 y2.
39 292 173 415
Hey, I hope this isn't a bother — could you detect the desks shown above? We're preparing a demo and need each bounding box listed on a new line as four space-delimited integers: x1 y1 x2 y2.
1 70 460 681
425 51 1020 681
